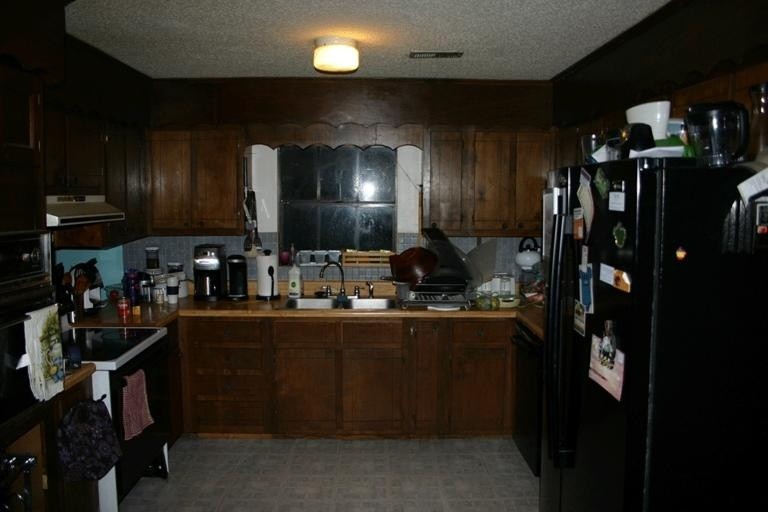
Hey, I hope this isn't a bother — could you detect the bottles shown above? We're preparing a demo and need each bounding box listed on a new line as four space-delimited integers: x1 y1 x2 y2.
166 277 179 305
142 284 151 303
156 288 164 304
118 297 130 318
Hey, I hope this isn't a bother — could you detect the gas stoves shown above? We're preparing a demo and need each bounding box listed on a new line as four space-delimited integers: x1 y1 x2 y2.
58 328 170 512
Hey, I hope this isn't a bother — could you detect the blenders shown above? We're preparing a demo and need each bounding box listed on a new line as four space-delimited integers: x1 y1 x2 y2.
255 249 281 301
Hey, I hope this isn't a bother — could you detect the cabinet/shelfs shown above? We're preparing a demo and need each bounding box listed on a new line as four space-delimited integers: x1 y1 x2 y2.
513 322 542 478
0 144 96 512
514 124 583 236
53 121 146 247
178 318 273 439
421 124 516 237
144 126 246 235
455 318 515 439
405 319 455 438
271 320 404 437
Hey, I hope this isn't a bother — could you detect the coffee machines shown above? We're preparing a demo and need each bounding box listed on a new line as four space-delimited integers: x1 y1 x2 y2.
193 243 229 302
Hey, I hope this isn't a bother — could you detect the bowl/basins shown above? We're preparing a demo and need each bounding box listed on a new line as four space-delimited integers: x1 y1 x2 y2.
399 246 437 281
627 101 670 140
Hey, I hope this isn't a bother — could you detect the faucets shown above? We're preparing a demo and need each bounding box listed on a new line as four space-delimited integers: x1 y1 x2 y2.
319 261 346 299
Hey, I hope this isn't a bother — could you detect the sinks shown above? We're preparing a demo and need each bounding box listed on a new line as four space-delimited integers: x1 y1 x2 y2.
285 297 340 314
340 298 398 310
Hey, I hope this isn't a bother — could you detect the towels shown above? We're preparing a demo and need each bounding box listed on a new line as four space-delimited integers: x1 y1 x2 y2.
122 370 154 442
23 304 65 402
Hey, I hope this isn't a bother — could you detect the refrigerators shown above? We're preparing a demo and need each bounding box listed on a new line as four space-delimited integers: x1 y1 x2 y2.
538 157 768 512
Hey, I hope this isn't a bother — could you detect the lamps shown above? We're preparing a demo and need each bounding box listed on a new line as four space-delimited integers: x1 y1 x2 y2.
312 36 361 73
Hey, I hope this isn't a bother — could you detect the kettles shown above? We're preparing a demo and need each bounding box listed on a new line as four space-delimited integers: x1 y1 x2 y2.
684 96 747 167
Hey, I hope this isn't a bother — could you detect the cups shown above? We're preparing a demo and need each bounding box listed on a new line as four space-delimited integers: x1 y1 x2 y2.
315 251 325 264
300 251 311 263
328 250 339 263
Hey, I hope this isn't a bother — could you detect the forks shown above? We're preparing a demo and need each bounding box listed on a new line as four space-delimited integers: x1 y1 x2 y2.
251 191 263 250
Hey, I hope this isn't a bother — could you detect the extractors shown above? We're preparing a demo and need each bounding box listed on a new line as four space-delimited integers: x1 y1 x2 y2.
46 194 125 227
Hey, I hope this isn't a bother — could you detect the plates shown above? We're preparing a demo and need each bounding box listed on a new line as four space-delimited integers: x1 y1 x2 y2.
297 299 333 309
352 298 388 310
427 305 461 311
